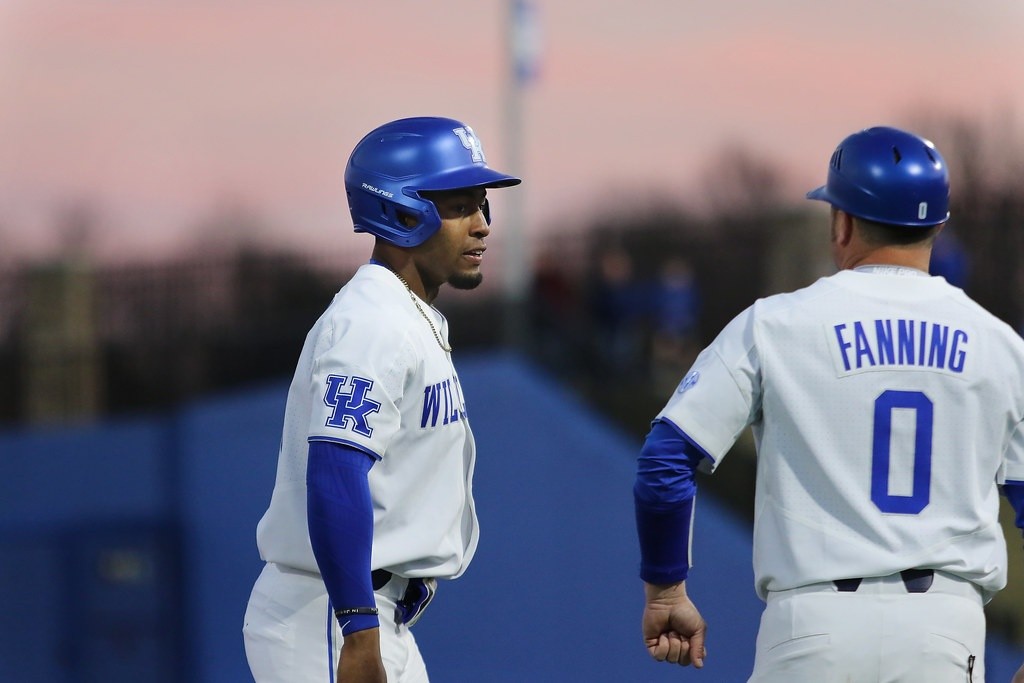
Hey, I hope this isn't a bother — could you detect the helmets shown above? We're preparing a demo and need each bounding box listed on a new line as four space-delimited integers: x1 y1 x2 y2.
806 126 951 225
344 117 522 247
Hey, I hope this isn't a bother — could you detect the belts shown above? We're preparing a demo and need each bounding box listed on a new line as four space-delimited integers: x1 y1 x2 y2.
372 568 420 604
832 568 934 593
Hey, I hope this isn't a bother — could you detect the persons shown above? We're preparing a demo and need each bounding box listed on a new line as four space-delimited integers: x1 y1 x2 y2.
632 127 1024 682
240 116 523 682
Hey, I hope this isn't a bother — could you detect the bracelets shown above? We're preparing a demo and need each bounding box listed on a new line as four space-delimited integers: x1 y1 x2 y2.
335 608 378 618
